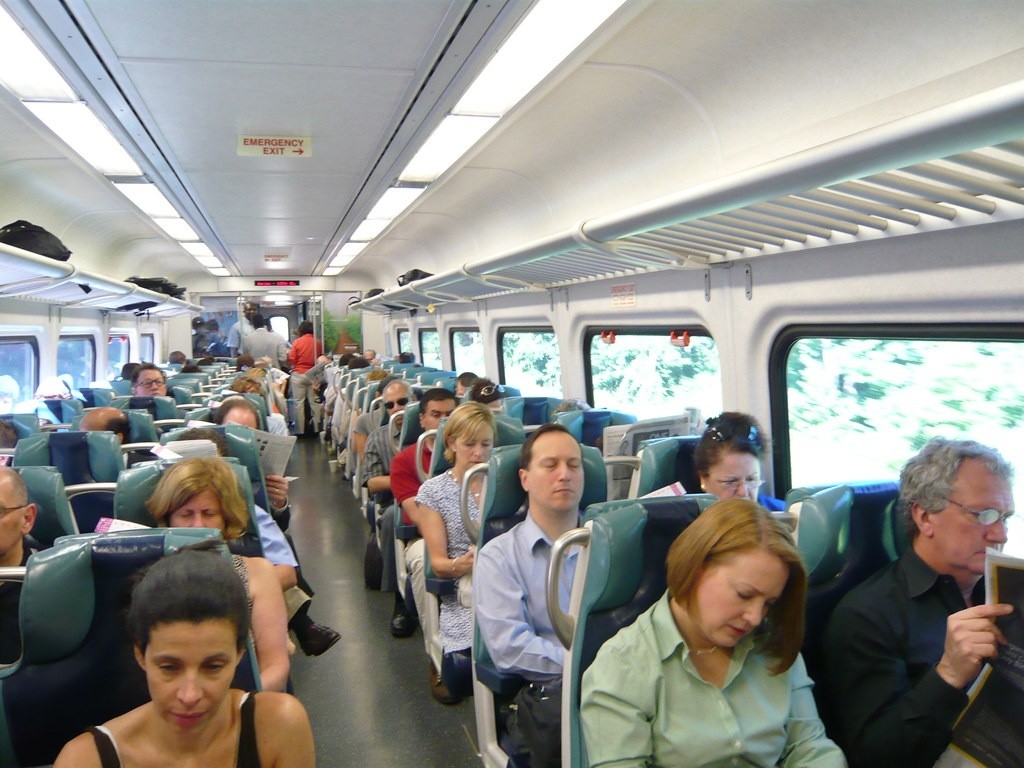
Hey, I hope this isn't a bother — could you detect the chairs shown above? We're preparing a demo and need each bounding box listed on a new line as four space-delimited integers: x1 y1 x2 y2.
0 356 912 768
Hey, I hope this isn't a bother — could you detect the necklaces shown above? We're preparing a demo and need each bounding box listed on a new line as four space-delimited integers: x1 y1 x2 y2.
448 471 480 498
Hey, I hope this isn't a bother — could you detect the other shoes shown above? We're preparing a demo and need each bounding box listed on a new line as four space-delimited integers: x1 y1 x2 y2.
430 672 454 704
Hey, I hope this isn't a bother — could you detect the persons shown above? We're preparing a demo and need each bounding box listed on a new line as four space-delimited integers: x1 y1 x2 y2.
214 399 342 657
288 320 322 439
180 427 299 593
226 302 258 357
312 349 418 637
474 423 584 768
0 421 17 467
580 498 848 768
52 538 316 768
415 401 499 657
455 371 504 411
192 317 232 357
826 436 1014 768
80 407 156 469
693 411 786 511
390 388 460 705
0 465 45 666
147 456 290 691
243 315 287 372
121 351 287 420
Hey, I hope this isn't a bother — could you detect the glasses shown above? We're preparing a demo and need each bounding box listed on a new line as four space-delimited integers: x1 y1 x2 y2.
478 384 507 396
940 494 1013 527
137 377 165 390
0 505 25 520
383 396 413 410
707 423 760 446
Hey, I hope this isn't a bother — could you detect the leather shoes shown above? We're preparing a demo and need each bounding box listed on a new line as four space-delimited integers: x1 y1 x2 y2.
298 622 342 657
391 600 414 638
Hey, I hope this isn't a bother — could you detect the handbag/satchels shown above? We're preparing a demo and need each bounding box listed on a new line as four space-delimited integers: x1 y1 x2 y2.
365 532 384 591
505 676 563 760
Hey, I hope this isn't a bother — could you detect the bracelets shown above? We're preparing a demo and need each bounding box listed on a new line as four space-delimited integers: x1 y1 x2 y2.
452 558 457 577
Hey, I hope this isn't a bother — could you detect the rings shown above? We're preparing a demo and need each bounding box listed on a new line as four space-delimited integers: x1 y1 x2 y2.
275 489 278 494
